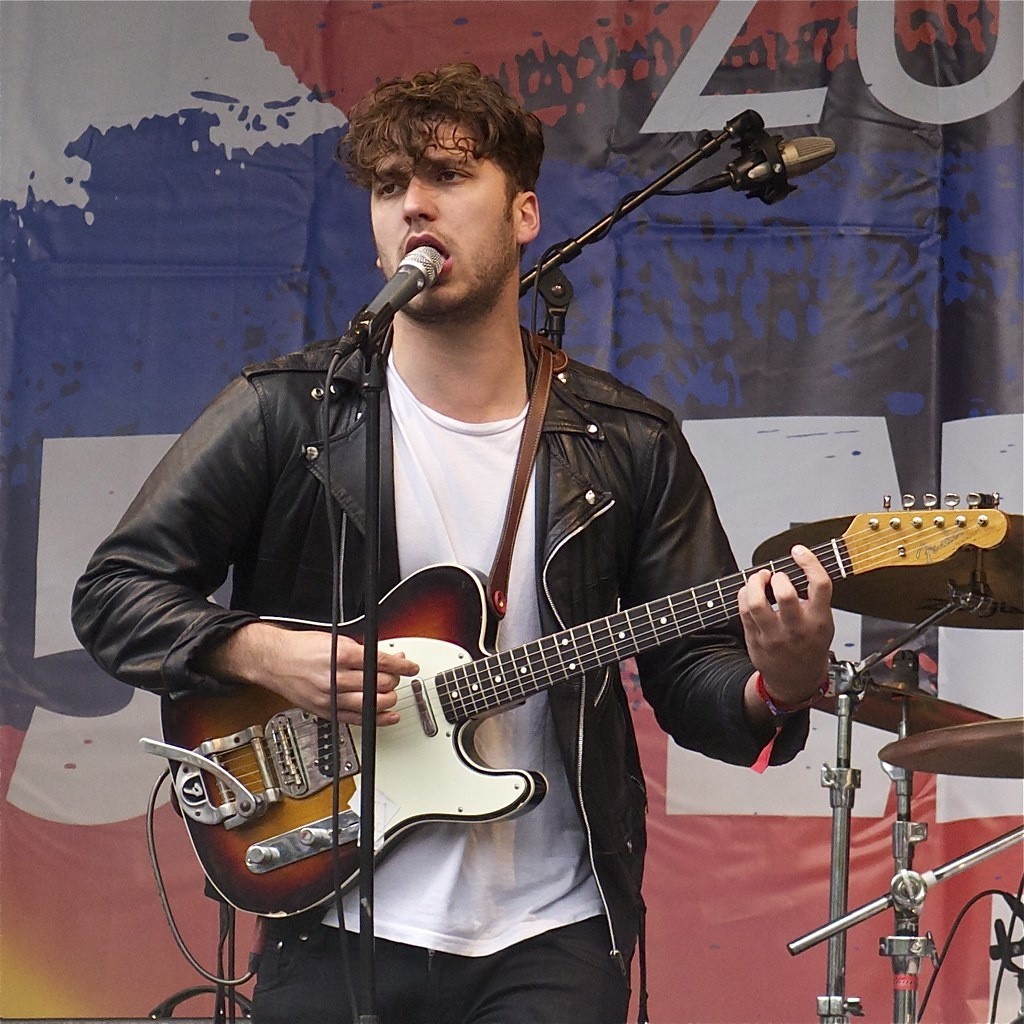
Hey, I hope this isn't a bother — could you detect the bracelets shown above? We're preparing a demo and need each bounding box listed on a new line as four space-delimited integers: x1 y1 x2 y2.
758 667 830 718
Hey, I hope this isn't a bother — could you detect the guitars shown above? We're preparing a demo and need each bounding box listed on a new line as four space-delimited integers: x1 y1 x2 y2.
154 488 1013 927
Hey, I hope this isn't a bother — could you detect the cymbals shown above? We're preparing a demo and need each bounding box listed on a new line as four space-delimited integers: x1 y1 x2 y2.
805 673 1004 735
752 509 1024 632
878 715 1024 779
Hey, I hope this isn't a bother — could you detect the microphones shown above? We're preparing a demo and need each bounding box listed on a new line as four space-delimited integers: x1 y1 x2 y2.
331 245 443 357
691 137 837 195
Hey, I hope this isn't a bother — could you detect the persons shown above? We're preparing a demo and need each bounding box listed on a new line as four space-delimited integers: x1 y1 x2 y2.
70 62 836 1024
